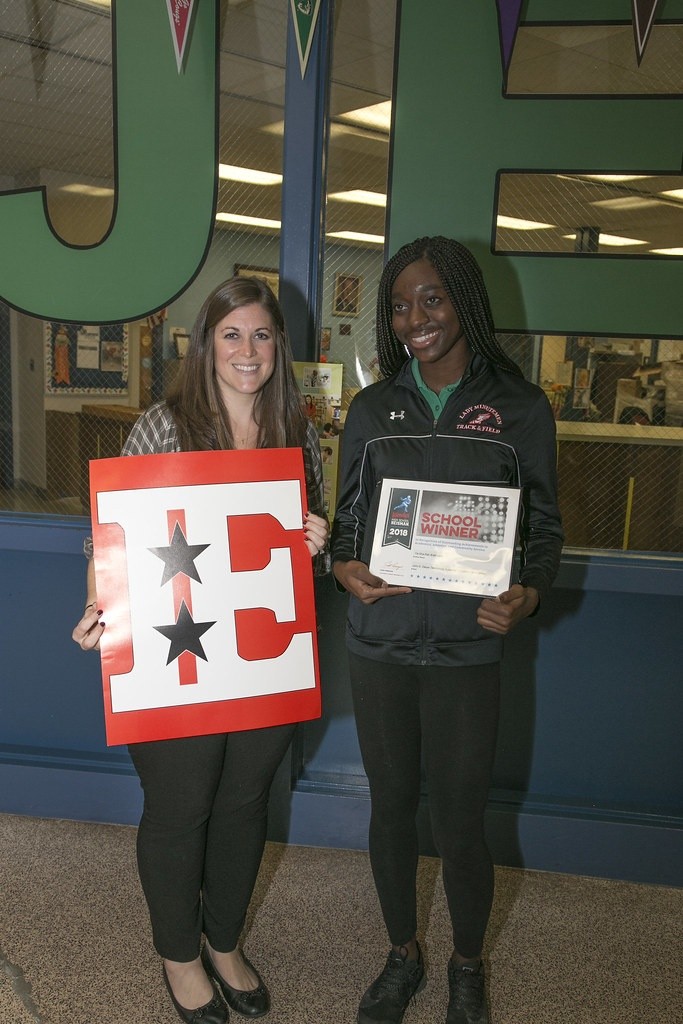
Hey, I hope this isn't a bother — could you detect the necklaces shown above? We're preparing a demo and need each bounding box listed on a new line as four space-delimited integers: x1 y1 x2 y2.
233 425 260 444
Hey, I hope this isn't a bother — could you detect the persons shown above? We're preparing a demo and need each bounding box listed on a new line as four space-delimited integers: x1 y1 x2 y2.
325 235 563 1024
337 278 357 313
302 370 340 465
72 273 329 1024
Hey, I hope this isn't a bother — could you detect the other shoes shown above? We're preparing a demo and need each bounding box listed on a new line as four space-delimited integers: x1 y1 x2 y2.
162 957 229 1024
201 943 271 1017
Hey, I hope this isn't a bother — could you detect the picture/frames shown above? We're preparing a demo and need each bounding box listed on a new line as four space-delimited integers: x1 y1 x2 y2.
233 262 279 301
339 324 351 336
332 272 362 318
320 327 331 351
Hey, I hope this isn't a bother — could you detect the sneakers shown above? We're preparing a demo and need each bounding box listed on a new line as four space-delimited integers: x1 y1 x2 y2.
445 958 488 1024
358 941 427 1024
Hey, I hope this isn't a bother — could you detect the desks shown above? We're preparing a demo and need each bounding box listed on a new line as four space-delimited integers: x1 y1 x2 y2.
79 404 145 515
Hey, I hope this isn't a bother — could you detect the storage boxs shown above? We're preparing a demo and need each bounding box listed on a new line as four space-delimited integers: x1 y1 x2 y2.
618 379 642 399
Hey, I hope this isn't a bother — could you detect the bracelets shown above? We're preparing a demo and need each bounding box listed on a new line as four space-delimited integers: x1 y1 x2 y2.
85 604 94 611
